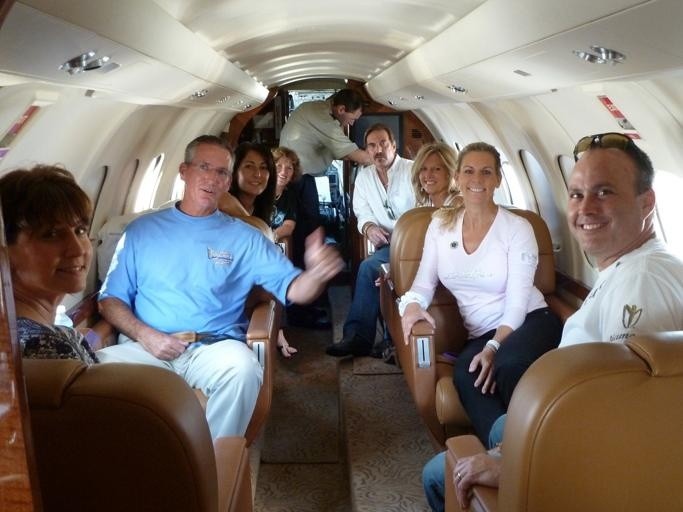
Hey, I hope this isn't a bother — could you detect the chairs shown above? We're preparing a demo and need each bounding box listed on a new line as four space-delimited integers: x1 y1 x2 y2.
378 205 445 318
21 356 252 508
442 331 680 508
233 215 271 444
400 211 578 416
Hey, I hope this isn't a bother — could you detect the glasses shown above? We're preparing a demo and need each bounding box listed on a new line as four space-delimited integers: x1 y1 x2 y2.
184 159 232 185
573 132 653 175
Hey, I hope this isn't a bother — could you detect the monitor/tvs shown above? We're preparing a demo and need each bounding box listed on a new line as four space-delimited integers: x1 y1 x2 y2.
354 111 404 158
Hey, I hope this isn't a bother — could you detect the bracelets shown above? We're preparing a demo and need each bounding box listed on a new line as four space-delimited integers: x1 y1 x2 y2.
362 221 377 239
485 338 502 350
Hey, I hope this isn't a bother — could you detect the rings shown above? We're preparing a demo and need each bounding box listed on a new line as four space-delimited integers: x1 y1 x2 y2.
457 473 465 482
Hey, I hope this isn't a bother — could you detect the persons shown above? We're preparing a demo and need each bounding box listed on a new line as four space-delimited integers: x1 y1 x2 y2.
327 122 411 365
409 141 464 209
1 162 102 366
265 148 301 363
394 142 566 444
418 129 681 511
275 86 374 327
86 131 347 449
230 140 276 222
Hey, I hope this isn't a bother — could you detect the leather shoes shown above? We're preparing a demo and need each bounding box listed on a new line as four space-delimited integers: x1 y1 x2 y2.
325 336 363 357
